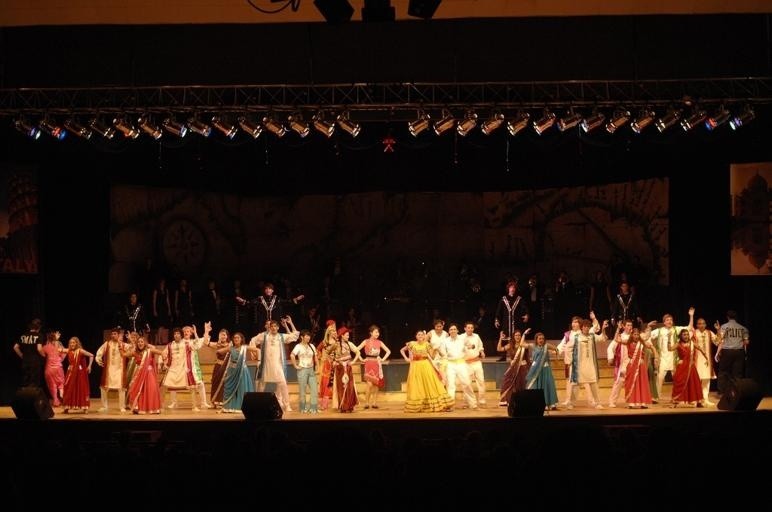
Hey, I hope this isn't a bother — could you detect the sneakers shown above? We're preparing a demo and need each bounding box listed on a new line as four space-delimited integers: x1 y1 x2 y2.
280 403 379 414
53 397 216 416
468 398 715 410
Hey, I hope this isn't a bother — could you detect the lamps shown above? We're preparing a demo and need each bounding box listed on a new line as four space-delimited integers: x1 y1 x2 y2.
10 98 757 142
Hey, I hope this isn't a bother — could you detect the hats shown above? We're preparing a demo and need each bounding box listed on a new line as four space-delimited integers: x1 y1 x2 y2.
335 326 353 337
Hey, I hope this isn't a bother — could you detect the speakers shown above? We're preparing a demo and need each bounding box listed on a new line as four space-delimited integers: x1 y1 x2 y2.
243 392 282 419
718 378 762 410
11 386 54 418
508 389 545 418
